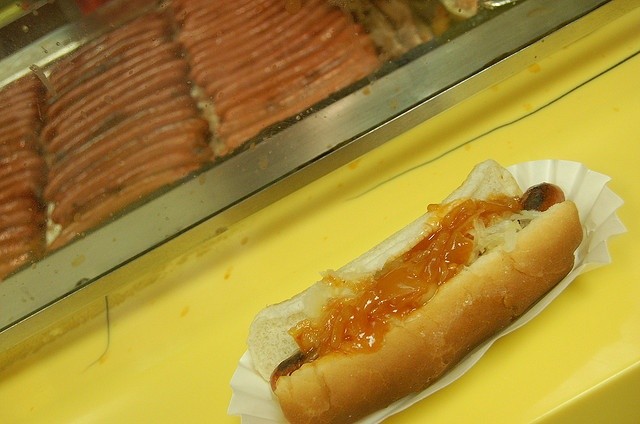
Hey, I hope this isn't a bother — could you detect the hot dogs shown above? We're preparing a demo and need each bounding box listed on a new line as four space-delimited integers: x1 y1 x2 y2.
247 159 584 424
